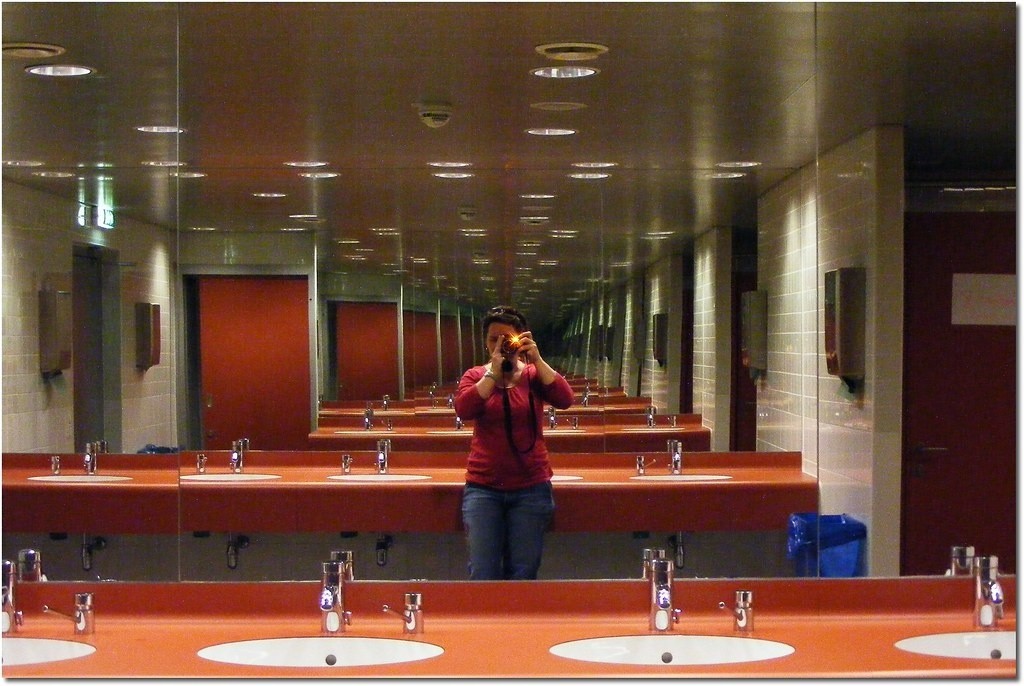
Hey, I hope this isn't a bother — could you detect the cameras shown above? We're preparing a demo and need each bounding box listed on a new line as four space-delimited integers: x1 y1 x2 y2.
500 337 527 354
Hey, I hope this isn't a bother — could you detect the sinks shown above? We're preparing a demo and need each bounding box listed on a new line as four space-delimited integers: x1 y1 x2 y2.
548 474 584 481
425 408 456 413
629 474 733 481
547 632 797 667
1 637 98 667
179 473 282 481
195 634 447 669
361 409 408 416
541 429 588 435
25 475 135 482
326 474 433 481
571 404 615 410
892 629 1017 660
618 427 687 432
332 430 397 435
426 429 474 436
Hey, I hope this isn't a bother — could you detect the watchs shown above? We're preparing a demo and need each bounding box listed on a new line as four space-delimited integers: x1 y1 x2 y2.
484 370 498 382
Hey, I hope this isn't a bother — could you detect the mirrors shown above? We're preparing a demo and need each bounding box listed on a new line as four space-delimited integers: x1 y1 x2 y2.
2 161 805 456
2 2 1016 582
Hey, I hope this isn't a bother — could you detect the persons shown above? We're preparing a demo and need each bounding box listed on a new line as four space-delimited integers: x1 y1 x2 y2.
453 304 573 582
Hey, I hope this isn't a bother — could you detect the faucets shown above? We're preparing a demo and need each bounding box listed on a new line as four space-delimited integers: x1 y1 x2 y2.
16 547 45 583
363 414 374 430
943 544 976 577
666 439 678 453
669 441 683 475
329 549 355 582
645 557 677 632
641 547 667 580
229 439 243 472
363 401 375 428
83 442 97 474
96 439 108 453
972 554 1007 628
645 405 656 427
579 391 589 406
376 440 389 473
455 413 465 430
547 406 558 429
240 437 250 451
446 391 456 408
1 558 16 633
318 559 350 635
381 394 391 411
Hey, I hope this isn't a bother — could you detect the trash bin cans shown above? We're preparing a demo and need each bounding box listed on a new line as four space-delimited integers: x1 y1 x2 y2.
789 514 866 579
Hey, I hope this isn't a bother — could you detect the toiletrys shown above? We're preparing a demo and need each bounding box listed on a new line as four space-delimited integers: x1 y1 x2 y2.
380 438 392 452
